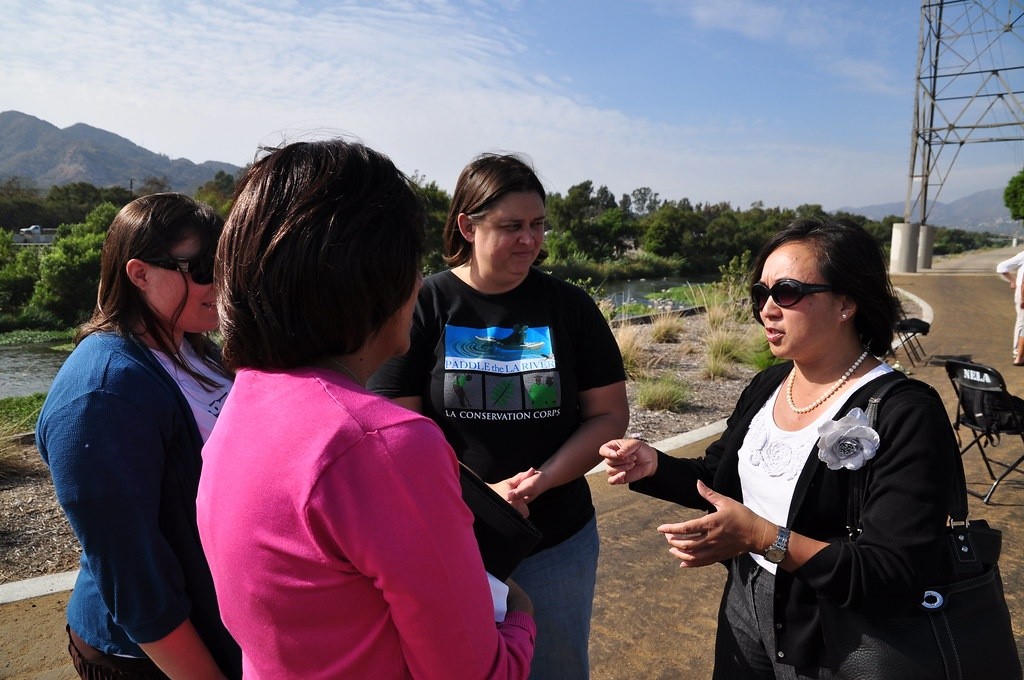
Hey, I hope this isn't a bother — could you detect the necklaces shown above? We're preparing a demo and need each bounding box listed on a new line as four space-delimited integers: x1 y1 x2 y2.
329 354 362 387
787 347 870 413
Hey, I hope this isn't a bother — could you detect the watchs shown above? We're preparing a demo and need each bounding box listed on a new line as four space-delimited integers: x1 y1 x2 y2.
763 524 792 565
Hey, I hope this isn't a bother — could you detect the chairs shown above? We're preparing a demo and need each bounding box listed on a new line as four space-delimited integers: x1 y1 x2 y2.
885 296 930 368
945 357 1024 504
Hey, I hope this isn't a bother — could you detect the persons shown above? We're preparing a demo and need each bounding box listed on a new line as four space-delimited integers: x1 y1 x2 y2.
598 217 967 680
196 138 537 680
36 193 242 680
996 249 1024 365
364 150 629 680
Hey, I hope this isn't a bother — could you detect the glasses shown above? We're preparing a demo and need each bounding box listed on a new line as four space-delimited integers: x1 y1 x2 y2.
138 251 216 285
750 279 834 310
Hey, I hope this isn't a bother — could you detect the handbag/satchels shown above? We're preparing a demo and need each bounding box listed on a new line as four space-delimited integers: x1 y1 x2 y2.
954 384 1024 447
846 379 1023 680
